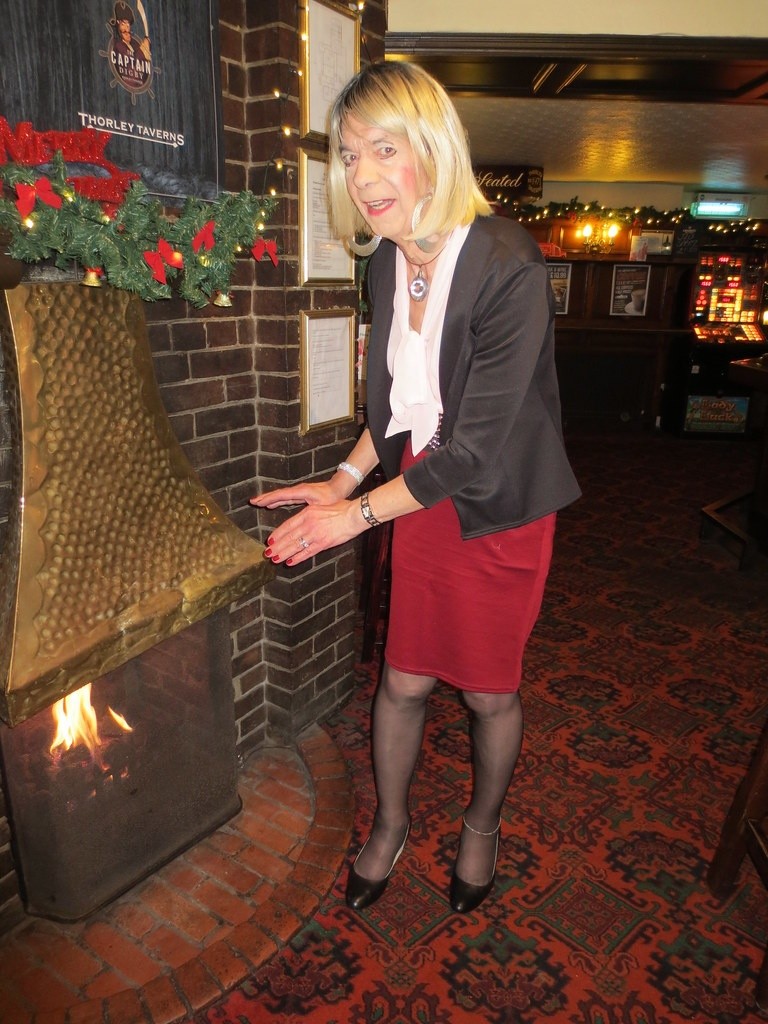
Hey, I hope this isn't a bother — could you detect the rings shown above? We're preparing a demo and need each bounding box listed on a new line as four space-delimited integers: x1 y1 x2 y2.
301 538 309 548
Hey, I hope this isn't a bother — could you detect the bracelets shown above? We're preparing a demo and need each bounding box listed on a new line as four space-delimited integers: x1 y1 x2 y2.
337 462 364 485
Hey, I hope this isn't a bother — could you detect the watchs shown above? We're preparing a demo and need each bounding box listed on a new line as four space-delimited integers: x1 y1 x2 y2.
360 492 381 527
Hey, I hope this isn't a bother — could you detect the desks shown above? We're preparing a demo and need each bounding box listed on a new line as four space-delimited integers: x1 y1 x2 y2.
697 353 768 568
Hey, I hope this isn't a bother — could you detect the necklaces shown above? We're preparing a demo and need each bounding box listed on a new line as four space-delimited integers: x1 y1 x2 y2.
400 231 454 302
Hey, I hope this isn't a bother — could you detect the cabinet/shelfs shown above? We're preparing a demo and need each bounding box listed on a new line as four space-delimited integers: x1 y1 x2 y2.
526 221 768 438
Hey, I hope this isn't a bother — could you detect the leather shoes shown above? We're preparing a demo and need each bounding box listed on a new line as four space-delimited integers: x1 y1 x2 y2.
450 826 500 914
346 820 411 910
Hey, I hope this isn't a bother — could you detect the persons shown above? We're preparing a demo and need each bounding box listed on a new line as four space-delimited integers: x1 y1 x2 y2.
250 62 556 917
